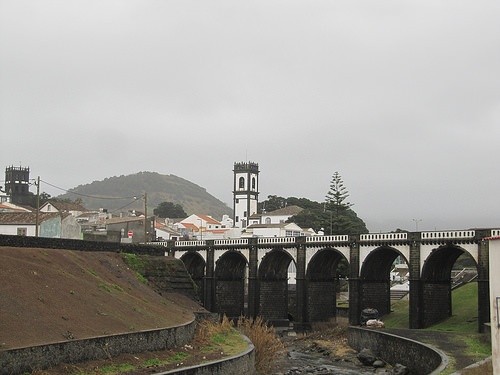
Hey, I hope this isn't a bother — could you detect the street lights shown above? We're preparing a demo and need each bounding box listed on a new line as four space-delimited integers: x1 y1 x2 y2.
197 219 202 245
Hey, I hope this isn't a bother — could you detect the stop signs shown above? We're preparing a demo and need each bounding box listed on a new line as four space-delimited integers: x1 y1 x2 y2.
128 231 133 236
